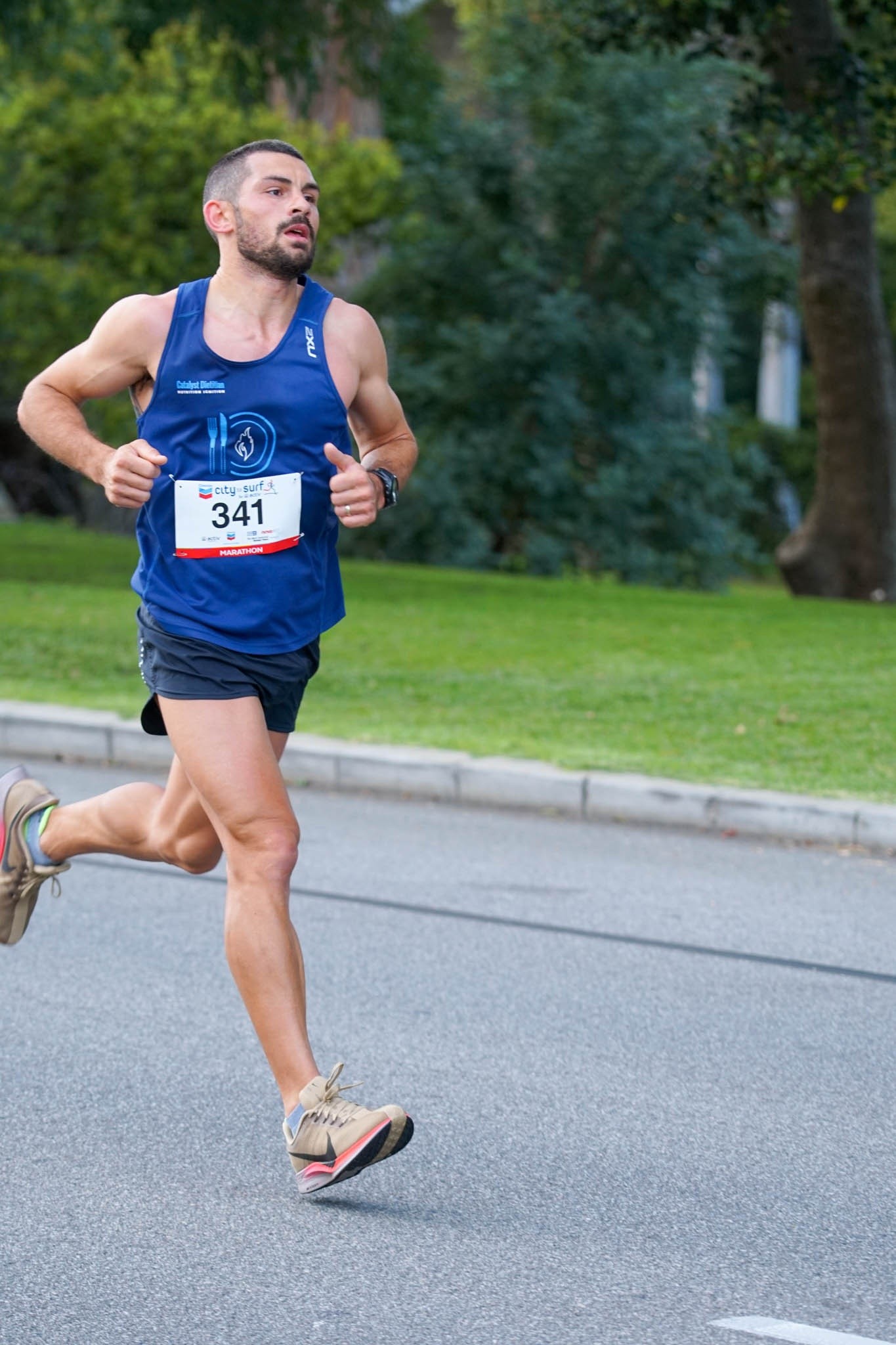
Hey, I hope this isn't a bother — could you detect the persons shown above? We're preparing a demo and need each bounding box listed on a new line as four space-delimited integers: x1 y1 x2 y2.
0 139 418 1196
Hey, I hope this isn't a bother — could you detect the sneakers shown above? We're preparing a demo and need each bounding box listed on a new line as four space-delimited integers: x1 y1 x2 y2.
0 762 72 947
281 1062 414 1194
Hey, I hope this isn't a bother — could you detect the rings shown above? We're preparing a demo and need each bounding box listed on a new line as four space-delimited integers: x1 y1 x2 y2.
345 505 353 517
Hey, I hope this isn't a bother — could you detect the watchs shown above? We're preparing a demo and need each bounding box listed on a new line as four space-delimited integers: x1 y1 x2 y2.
366 466 400 510
